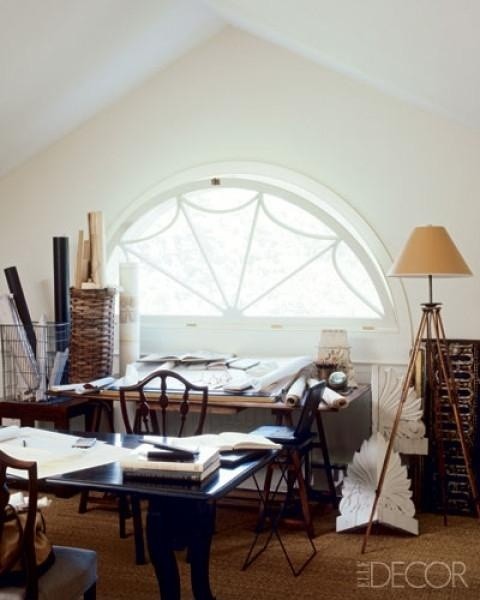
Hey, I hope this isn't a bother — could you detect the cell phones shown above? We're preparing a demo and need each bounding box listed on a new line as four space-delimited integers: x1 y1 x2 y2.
147 452 193 460
72 436 97 448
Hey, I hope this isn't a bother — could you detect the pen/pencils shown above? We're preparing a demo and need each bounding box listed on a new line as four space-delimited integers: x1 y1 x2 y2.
138 439 199 459
23 440 25 447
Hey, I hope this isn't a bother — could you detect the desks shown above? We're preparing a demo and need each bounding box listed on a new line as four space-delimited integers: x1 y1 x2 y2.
43 381 371 539
0 428 284 600
0 393 113 500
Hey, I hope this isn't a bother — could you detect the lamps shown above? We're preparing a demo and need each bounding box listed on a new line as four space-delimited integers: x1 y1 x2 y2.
387 225 474 303
118 261 139 376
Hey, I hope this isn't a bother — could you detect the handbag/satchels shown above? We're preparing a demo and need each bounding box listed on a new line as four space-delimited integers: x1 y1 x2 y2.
0 504 55 587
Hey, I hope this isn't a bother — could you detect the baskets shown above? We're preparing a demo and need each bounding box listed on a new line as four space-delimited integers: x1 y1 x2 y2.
0 321 69 405
69 286 116 384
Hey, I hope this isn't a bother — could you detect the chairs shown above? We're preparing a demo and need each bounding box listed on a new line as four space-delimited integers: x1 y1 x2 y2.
239 379 326 575
0 449 98 600
115 370 208 565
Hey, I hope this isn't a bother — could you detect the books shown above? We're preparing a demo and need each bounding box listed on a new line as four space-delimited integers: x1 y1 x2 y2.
120 432 284 481
